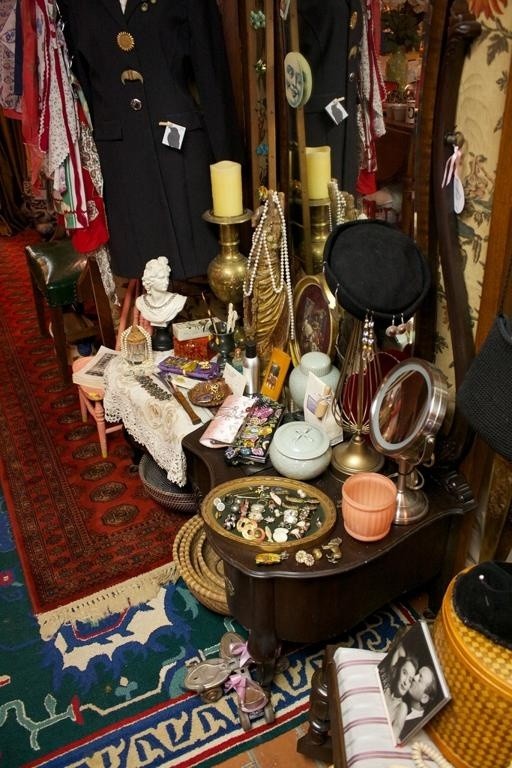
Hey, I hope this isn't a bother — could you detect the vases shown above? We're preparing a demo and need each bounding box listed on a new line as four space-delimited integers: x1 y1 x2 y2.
340 471 398 543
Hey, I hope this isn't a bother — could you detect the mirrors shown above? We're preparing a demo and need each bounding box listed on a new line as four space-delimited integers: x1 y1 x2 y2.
278 1 450 358
368 358 448 525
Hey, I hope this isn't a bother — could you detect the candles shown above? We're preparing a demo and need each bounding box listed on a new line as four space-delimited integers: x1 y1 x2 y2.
210 161 243 217
304 146 331 199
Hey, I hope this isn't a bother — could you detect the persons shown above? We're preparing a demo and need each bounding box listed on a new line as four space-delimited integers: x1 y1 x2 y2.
400 662 439 741
383 643 417 737
284 53 307 106
133 256 187 321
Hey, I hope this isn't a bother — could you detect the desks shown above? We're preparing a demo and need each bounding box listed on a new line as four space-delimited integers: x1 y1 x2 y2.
374 119 416 242
103 350 476 688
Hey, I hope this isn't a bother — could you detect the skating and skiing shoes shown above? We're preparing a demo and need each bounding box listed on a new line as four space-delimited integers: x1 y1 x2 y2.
184 646 283 702
221 632 274 729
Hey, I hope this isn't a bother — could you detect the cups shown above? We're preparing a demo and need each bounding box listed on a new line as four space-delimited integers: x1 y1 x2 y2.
341 471 398 542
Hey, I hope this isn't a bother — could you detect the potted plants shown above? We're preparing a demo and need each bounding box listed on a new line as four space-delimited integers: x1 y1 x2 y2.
379 2 425 93
383 89 408 121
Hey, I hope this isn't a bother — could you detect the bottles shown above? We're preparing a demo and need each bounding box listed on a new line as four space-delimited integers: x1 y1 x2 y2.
243 342 260 395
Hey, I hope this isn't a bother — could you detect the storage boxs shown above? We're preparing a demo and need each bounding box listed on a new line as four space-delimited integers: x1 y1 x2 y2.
171 317 220 360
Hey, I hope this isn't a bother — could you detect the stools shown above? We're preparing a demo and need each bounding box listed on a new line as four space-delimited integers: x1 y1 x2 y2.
296 643 443 768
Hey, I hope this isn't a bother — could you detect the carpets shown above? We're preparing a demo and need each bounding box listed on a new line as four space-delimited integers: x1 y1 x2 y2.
0 217 422 768
0 229 182 641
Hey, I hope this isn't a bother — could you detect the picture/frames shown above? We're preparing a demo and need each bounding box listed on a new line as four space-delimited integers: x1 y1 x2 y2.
286 274 339 368
261 347 292 401
374 617 451 747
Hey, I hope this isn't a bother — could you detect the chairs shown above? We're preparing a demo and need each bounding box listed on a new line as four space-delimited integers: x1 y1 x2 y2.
25 216 89 387
72 279 153 458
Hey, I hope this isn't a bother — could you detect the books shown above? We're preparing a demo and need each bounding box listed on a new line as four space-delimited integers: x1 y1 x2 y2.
376 619 450 746
72 344 121 389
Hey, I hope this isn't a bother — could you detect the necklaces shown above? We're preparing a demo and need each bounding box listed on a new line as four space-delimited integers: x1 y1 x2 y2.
330 180 348 226
117 324 157 363
242 190 296 342
